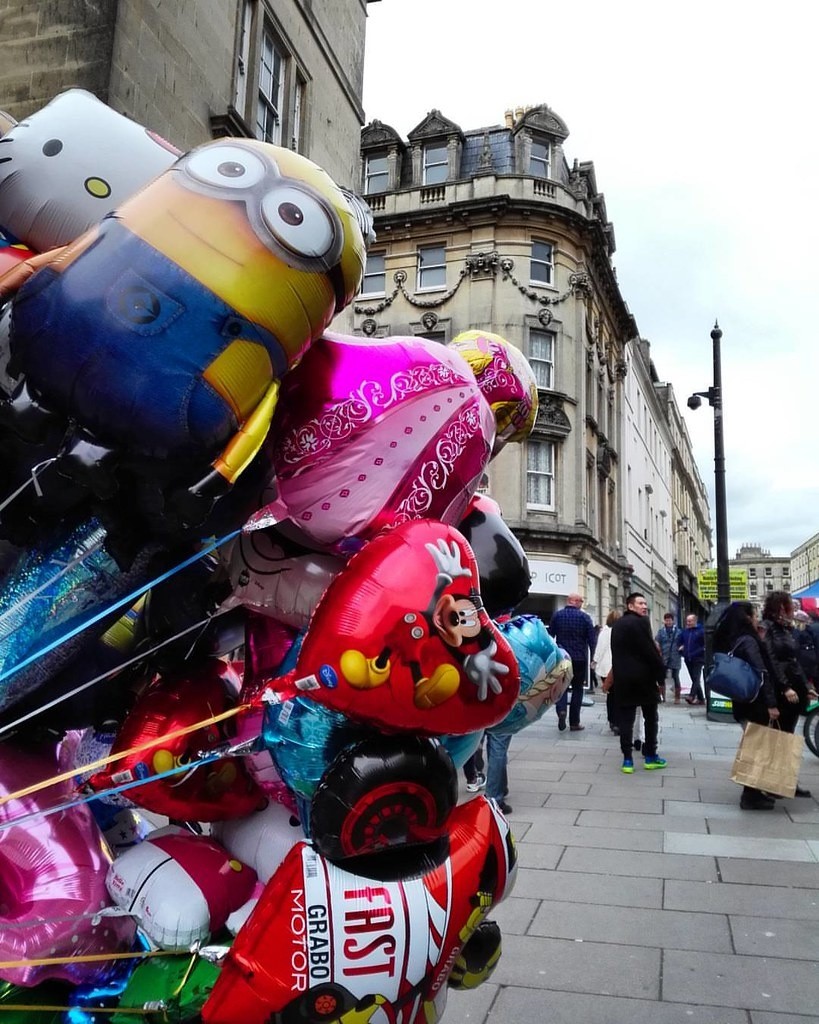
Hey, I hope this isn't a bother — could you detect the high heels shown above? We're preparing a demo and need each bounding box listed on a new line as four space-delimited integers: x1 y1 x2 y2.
612 726 619 736
634 738 642 750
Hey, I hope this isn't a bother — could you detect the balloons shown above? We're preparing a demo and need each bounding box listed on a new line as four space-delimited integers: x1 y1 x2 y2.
0 86 574 1023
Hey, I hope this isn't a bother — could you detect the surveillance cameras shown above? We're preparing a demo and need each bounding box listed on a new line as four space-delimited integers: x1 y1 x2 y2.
687 396 701 410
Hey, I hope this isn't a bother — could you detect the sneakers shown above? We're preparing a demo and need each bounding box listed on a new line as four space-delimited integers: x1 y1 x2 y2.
621 759 634 773
467 773 486 792
644 755 668 770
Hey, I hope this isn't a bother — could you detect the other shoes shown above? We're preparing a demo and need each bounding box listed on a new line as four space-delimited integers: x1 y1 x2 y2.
500 803 513 814
685 695 693 704
571 724 584 730
594 679 598 687
558 710 566 730
692 700 704 705
797 787 811 797
741 792 775 810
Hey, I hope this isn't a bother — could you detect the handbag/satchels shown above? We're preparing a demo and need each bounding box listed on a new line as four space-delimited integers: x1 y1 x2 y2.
662 655 671 666
731 720 804 799
705 635 762 703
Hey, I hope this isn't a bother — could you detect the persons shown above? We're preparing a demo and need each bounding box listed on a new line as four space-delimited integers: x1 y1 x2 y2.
654 613 682 705
484 732 513 815
611 592 668 773
593 624 605 688
548 592 597 732
593 610 645 750
463 737 487 793
762 590 810 799
708 601 779 811
794 608 819 702
677 614 705 705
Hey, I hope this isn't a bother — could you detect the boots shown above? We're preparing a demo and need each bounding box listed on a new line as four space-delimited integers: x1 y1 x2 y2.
674 688 680 704
660 686 666 702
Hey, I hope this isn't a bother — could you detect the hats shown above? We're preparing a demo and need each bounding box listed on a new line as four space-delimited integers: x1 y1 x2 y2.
794 610 809 622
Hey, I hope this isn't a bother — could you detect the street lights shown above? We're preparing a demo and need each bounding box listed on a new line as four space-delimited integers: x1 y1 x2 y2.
688 317 731 603
